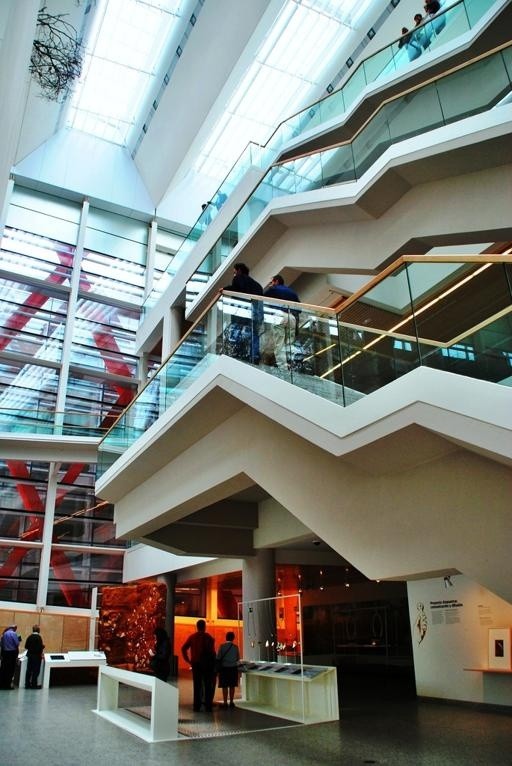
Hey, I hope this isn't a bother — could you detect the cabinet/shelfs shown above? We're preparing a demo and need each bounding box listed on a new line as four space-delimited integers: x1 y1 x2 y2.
41 646 111 692
228 656 340 727
91 662 181 748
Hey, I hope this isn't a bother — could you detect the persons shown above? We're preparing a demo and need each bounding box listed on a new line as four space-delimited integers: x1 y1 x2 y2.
181 618 216 713
1 628 10 689
398 25 422 58
200 203 213 226
220 262 262 366
23 623 44 689
149 626 170 683
218 631 241 709
207 188 227 210
203 636 218 708
425 0 446 34
2 622 20 690
262 276 301 377
413 13 431 49
